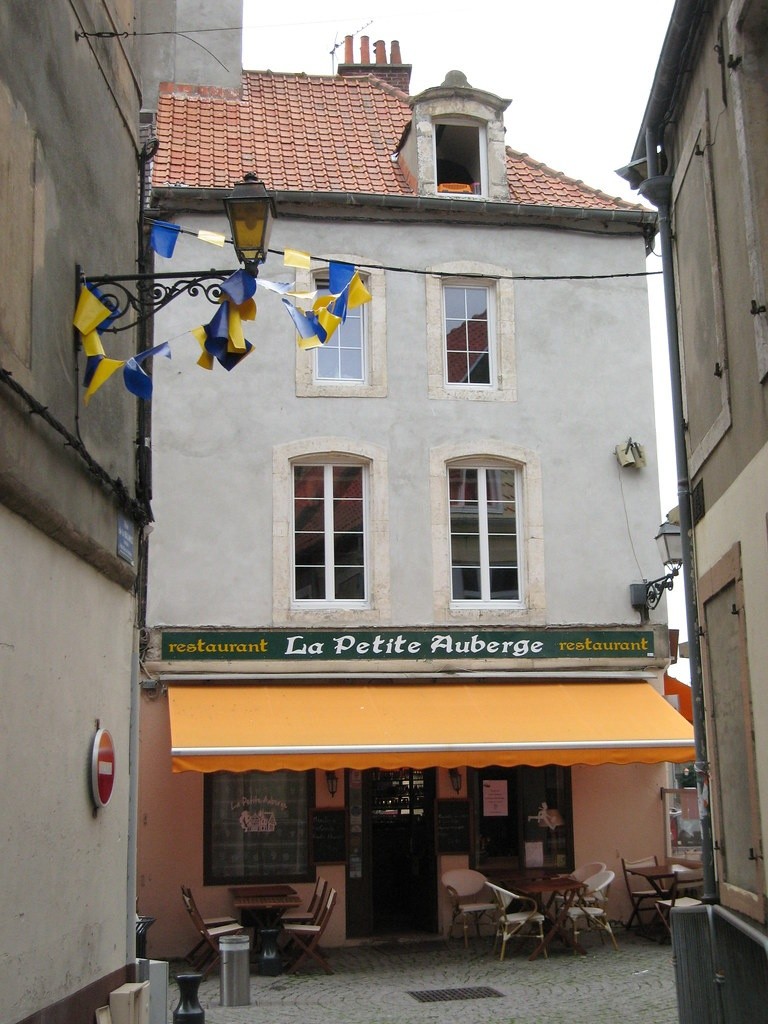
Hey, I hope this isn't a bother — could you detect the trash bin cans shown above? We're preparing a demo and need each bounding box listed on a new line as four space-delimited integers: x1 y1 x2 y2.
219 935 250 1006
136 916 157 959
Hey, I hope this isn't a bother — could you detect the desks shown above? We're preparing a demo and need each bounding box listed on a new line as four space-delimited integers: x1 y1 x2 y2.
228 885 298 952
232 896 305 975
499 871 554 935
512 878 590 960
625 866 700 941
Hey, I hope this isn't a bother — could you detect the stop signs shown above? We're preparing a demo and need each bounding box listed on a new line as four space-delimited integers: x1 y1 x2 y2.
91 727 118 808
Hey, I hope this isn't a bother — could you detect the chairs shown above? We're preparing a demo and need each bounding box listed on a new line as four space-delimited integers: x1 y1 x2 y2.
622 857 669 933
557 871 619 956
484 882 549 960
279 888 338 975
441 867 503 949
653 869 704 944
181 886 239 966
183 895 245 982
276 877 328 964
552 861 606 945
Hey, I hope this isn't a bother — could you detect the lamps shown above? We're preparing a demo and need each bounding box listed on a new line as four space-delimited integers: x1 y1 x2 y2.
448 768 462 795
641 521 682 623
324 770 338 798
74 172 278 354
